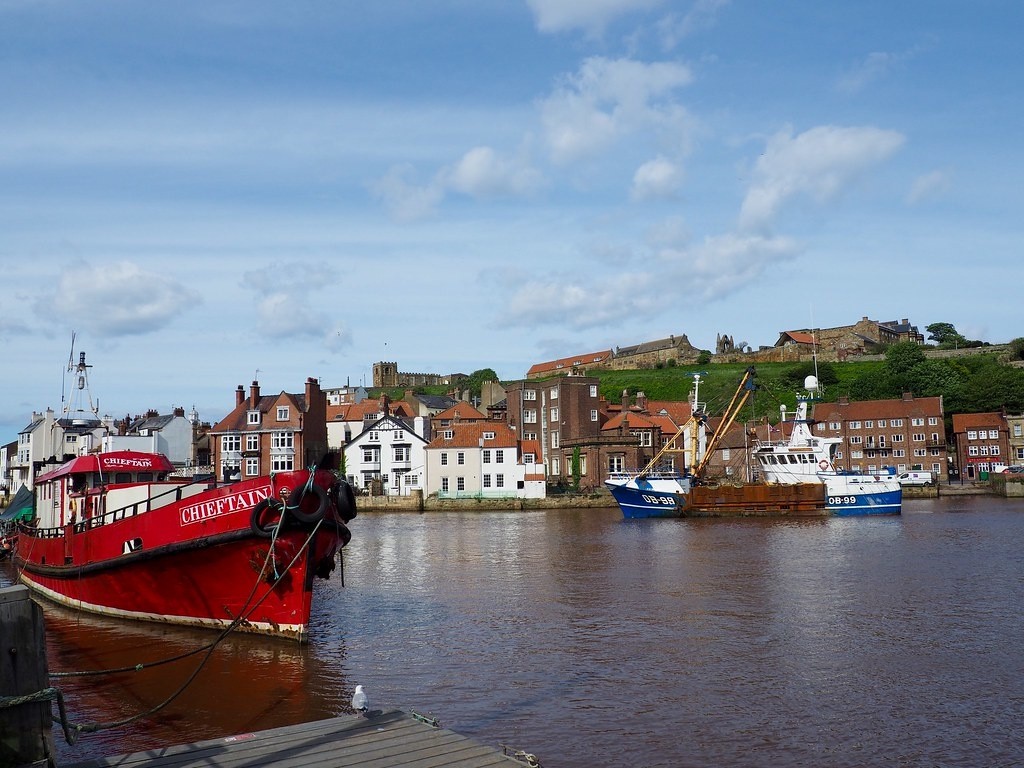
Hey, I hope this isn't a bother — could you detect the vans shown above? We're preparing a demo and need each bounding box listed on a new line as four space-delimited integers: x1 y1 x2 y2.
896 472 932 487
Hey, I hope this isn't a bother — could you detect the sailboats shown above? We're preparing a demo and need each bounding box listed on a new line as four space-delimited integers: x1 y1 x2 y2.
678 362 835 519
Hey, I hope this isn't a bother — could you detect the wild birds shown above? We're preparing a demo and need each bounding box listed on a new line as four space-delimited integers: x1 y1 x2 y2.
352 685 369 714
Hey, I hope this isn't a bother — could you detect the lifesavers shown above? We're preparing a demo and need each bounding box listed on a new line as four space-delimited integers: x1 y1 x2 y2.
335 482 358 521
289 482 329 523
250 498 286 538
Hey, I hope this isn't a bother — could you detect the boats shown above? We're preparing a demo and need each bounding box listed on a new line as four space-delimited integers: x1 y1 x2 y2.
603 371 903 520
11 450 358 645
